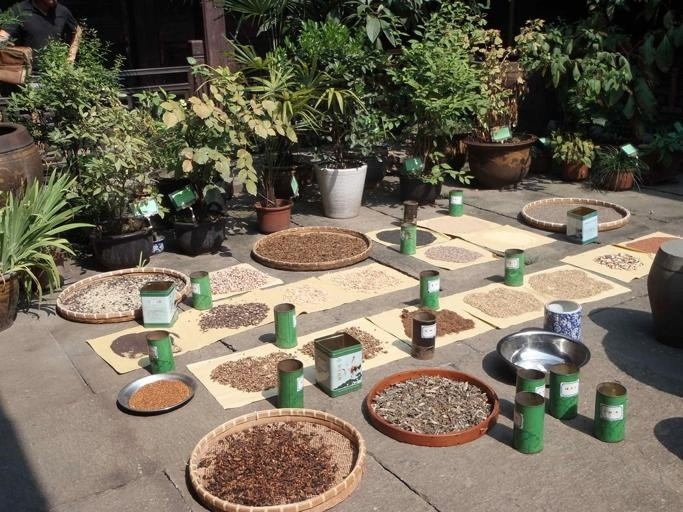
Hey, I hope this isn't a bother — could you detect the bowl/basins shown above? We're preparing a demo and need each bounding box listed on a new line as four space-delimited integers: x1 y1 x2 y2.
496 330 589 385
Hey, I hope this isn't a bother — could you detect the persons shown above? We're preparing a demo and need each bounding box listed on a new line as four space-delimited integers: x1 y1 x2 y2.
0 1 83 67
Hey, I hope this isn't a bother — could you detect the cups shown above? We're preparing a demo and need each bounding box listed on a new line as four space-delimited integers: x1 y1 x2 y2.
594 382 626 442
548 363 581 420
513 369 545 455
546 301 581 338
399 198 418 255
147 329 174 375
273 302 297 348
277 359 305 408
502 247 527 286
190 269 213 311
449 190 463 217
414 268 439 359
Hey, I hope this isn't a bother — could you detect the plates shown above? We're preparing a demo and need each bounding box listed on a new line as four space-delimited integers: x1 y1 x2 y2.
117 373 197 413
